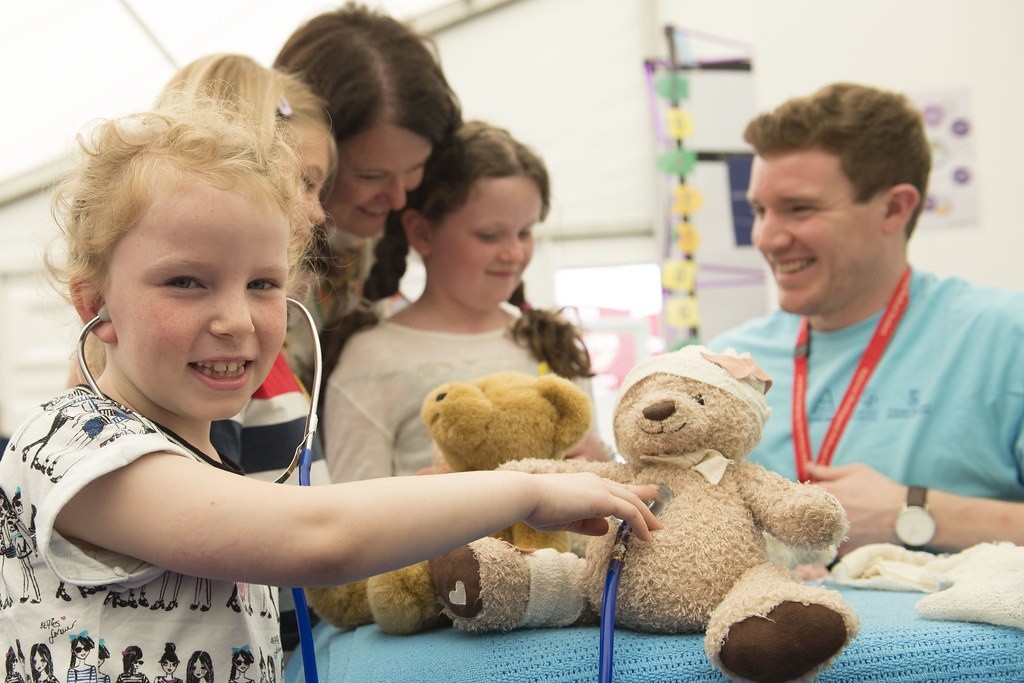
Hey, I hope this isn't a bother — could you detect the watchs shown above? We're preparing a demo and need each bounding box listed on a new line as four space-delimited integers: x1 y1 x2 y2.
893 484 937 552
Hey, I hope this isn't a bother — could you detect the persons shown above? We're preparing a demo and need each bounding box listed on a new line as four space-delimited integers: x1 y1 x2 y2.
140 54 340 663
273 3 466 416
320 119 614 489
695 83 1024 570
0 80 664 683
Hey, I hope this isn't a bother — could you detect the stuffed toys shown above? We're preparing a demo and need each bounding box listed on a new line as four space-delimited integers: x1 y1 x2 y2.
424 343 861 683
307 370 594 637
